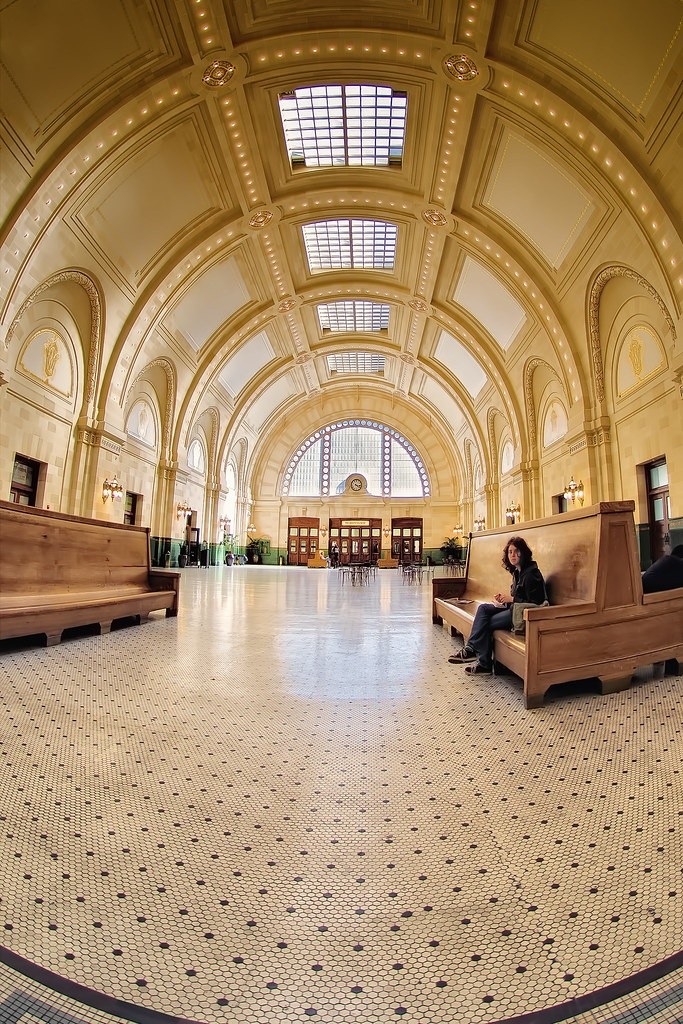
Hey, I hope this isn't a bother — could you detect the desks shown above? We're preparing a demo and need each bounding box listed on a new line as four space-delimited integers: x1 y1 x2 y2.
348 563 364 586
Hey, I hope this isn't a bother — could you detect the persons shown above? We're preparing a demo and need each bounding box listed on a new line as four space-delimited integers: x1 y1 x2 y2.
447 536 547 676
641 544 683 596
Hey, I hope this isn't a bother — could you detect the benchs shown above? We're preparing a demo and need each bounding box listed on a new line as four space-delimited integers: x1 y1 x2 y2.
377 550 399 569
308 550 327 568
432 500 683 710
0 499 182 646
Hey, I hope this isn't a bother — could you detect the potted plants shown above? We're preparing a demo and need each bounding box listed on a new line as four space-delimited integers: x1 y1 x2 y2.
441 536 462 559
220 533 240 554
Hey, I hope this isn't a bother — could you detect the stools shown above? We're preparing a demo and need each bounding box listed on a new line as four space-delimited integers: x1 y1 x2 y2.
442 557 466 578
398 564 436 586
338 569 375 587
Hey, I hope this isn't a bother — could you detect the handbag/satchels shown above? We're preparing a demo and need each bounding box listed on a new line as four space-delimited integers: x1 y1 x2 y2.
513 601 549 635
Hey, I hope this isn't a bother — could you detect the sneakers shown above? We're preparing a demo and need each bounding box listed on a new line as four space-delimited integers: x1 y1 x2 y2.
465 661 493 676
448 646 477 664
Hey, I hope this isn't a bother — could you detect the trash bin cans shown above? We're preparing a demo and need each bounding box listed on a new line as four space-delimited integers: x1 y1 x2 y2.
427 556 429 566
162 554 170 568
280 555 282 565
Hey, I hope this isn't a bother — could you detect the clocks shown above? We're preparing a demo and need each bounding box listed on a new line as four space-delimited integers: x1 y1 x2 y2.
351 479 362 491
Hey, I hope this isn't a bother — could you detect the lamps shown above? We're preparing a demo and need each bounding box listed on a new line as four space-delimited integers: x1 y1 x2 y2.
102 475 124 504
505 501 520 522
220 514 231 532
177 500 192 521
475 515 485 532
564 476 584 507
